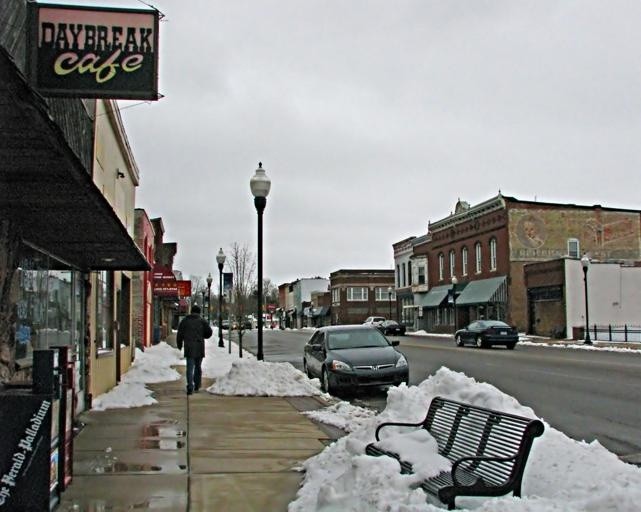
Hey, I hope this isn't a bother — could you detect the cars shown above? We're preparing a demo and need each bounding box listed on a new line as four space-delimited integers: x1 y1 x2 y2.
303 323 409 396
455 319 519 350
213 315 258 330
377 319 407 336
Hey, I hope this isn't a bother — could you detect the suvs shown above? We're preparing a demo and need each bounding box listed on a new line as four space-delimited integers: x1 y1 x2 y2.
363 316 386 326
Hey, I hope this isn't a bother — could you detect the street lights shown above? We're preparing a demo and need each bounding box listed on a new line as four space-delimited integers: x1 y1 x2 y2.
311 301 315 326
452 275 459 336
281 308 284 329
217 246 227 348
250 161 272 362
207 272 213 327
271 311 273 328
580 252 594 345
388 286 393 319
202 284 207 318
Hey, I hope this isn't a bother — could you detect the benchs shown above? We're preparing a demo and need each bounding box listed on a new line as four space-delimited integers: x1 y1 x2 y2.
366 392 545 511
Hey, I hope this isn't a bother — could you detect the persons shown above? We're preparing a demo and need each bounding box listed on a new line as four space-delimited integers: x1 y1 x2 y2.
177 306 212 394
